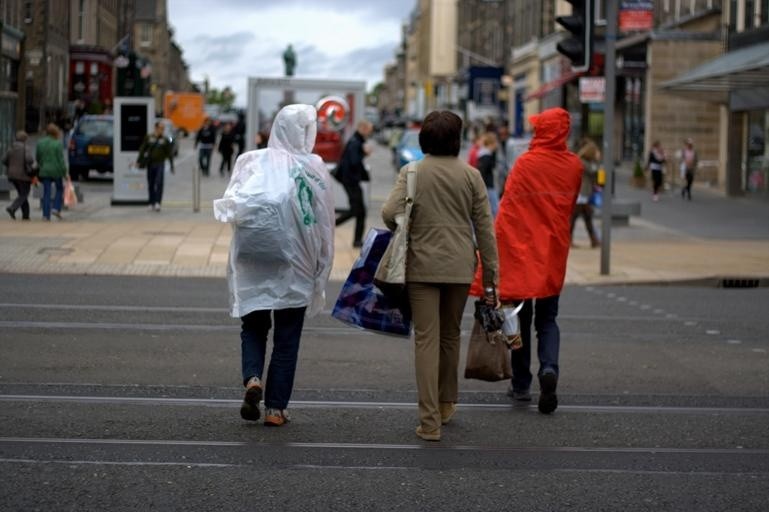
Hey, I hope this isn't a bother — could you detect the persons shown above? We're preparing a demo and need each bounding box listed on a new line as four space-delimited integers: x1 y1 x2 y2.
645 136 668 202
132 123 175 210
333 112 608 250
381 110 501 439
679 140 698 200
283 44 297 76
214 103 334 427
192 107 247 176
468 108 585 415
1 101 85 222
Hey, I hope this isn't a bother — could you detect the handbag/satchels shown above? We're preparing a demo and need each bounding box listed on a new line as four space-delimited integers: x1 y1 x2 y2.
139 152 150 167
465 320 513 381
331 228 411 336
374 224 412 294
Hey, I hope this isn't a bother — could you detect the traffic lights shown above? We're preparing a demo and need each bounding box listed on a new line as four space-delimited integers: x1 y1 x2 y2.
556 1 595 74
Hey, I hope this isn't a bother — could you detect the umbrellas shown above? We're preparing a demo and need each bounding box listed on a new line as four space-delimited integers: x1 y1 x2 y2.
475 286 505 333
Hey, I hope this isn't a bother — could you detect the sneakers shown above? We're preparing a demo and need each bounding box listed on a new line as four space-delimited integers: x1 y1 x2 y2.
147 202 160 211
240 376 262 421
439 401 457 424
416 425 440 440
508 383 531 401
539 374 557 413
264 408 291 425
7 207 61 221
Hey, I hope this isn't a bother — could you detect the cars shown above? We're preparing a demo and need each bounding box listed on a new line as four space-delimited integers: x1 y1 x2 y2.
157 118 180 156
68 114 115 182
396 130 425 170
